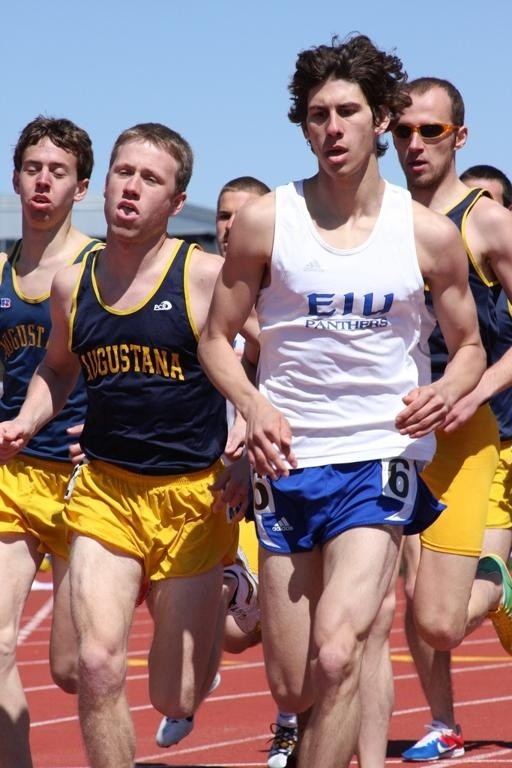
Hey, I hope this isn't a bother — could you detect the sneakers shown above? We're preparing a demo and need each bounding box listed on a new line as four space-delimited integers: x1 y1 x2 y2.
224 547 260 635
155 672 221 748
267 713 299 768
477 552 512 659
400 719 466 761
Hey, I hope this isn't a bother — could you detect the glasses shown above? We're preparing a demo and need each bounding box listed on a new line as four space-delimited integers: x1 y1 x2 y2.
394 121 458 141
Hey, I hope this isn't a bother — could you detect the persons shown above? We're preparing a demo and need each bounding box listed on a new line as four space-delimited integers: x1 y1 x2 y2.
0 113 89 768
458 161 512 569
193 28 486 768
352 73 512 768
212 170 270 652
1 120 262 768
